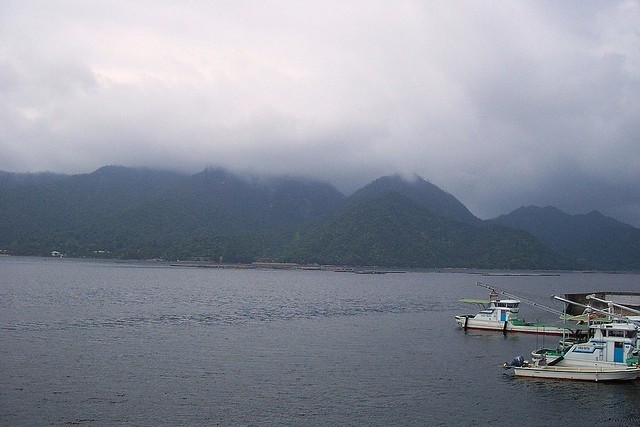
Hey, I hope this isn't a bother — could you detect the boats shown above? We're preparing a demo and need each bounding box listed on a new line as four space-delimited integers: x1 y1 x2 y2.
557 314 640 349
531 327 638 360
499 343 640 381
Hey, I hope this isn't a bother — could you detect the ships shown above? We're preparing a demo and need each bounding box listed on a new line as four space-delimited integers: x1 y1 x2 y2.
454 279 640 338
